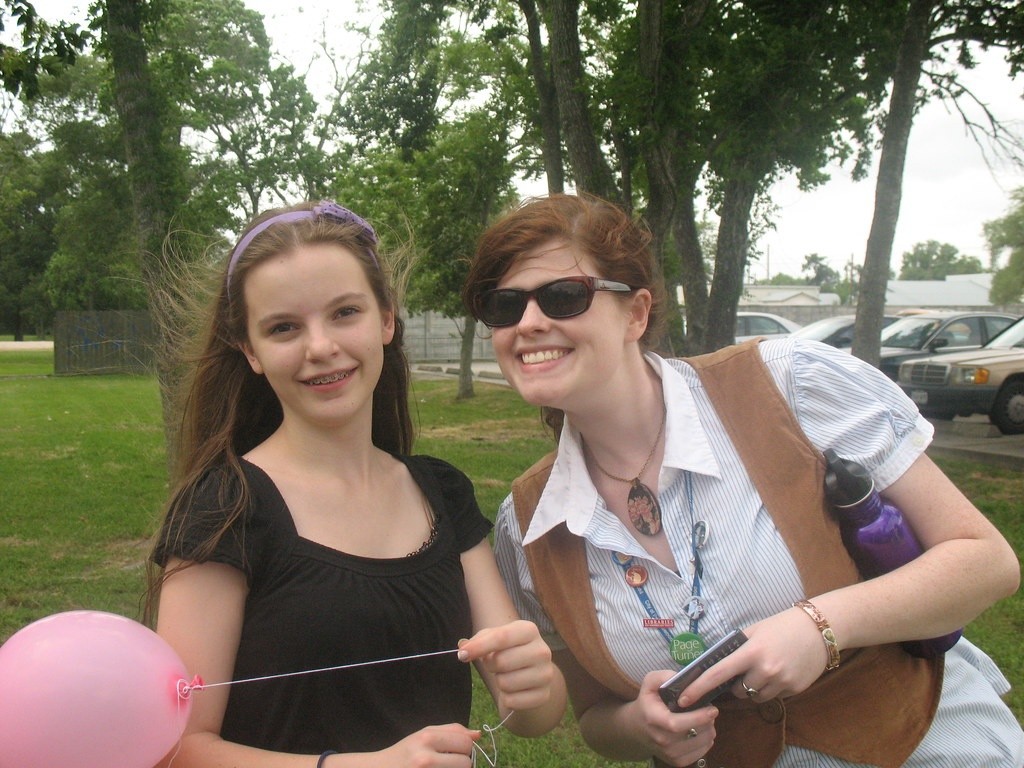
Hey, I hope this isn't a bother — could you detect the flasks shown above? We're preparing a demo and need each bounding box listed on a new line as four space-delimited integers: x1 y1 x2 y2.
823 449 962 657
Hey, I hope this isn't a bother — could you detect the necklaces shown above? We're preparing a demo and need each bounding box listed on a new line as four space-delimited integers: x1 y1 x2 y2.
578 405 666 536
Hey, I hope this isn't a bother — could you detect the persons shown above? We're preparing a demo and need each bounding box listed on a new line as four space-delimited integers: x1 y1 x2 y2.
458 189 1023 768
143 195 567 768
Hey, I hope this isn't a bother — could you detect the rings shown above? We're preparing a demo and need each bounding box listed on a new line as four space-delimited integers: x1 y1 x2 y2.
741 676 759 698
688 727 698 739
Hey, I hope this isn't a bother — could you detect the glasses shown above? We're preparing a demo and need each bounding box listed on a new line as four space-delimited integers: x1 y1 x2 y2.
472 275 639 327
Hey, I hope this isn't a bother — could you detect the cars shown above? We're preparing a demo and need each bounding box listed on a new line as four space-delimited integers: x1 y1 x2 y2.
734 311 797 342
841 310 1024 382
897 310 1024 433
789 315 898 348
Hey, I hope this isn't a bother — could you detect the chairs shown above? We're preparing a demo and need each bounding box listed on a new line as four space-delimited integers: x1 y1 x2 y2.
936 331 955 348
966 330 981 346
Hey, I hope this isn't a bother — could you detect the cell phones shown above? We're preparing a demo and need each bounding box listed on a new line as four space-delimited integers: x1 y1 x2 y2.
658 628 750 712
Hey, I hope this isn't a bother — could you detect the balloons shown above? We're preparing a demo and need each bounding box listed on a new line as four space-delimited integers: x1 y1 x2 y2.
0 610 204 768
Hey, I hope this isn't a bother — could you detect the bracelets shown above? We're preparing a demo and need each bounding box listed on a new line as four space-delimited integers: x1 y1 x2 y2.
317 750 338 768
792 599 840 671
